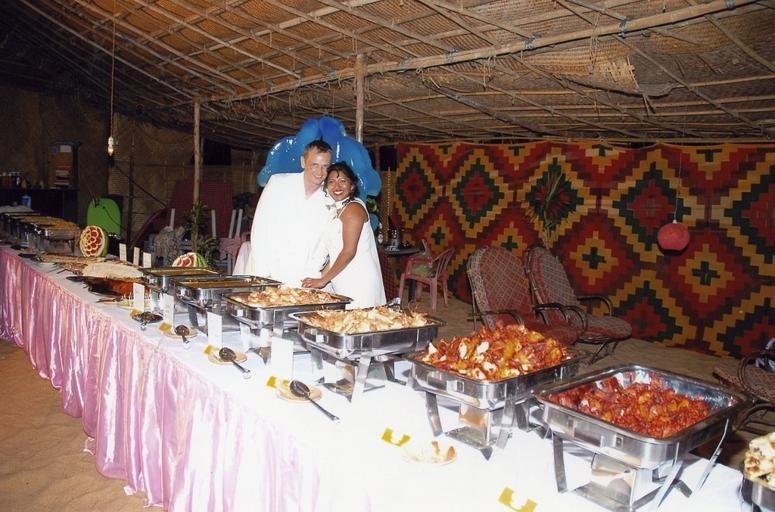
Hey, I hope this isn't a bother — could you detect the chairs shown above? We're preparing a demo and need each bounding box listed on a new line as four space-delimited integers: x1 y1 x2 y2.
705 335 775 442
395 244 456 314
463 244 581 393
522 245 631 366
142 206 251 277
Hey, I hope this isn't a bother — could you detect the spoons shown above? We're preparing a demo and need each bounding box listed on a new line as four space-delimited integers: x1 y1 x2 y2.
173 322 191 347
149 312 154 333
219 346 251 376
290 378 340 424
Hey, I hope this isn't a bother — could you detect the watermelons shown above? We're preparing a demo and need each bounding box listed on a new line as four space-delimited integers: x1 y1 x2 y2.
79 225 109 259
172 252 208 269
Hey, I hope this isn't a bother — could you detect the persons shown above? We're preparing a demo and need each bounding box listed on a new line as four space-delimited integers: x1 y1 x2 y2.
244 140 334 295
301 160 387 310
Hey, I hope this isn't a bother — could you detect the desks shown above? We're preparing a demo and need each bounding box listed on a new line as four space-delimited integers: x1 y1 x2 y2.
377 242 421 304
0 231 775 512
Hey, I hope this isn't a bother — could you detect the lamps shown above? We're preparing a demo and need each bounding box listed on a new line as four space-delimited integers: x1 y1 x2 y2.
657 101 692 253
105 0 117 158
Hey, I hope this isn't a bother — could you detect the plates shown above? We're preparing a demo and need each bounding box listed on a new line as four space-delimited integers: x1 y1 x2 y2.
276 384 323 403
130 311 165 323
208 350 247 366
411 441 457 465
163 325 199 340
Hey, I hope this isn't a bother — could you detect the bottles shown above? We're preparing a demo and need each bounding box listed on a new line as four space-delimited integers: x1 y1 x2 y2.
0 168 23 188
377 222 384 248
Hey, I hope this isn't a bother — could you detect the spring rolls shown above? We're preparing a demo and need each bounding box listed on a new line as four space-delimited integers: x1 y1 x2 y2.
745 432 775 486
